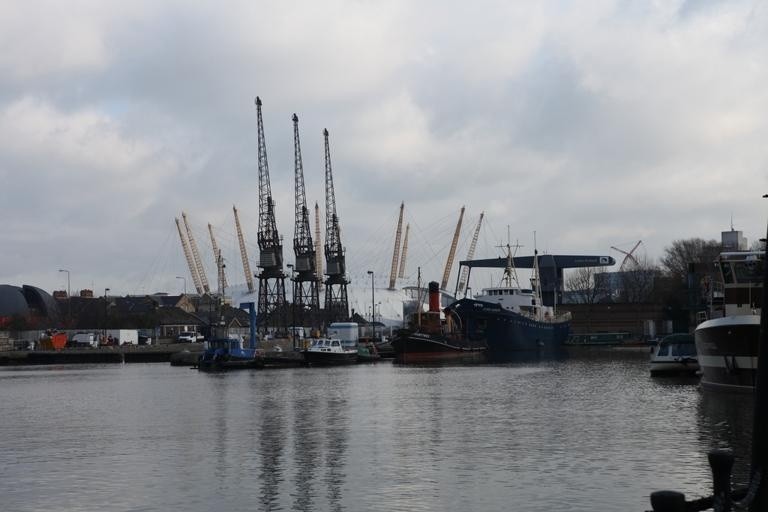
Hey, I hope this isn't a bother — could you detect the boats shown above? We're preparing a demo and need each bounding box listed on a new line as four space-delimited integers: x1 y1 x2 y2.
198 338 264 370
390 332 490 364
650 333 704 376
444 225 572 362
303 339 358 367
694 250 761 395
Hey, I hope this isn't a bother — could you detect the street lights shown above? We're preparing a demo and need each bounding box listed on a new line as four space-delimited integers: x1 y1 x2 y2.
368 271 374 342
287 264 295 350
176 277 186 312
105 288 110 329
60 270 72 329
763 194 768 286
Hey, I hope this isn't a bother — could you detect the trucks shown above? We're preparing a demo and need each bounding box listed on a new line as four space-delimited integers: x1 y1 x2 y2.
178 331 204 342
